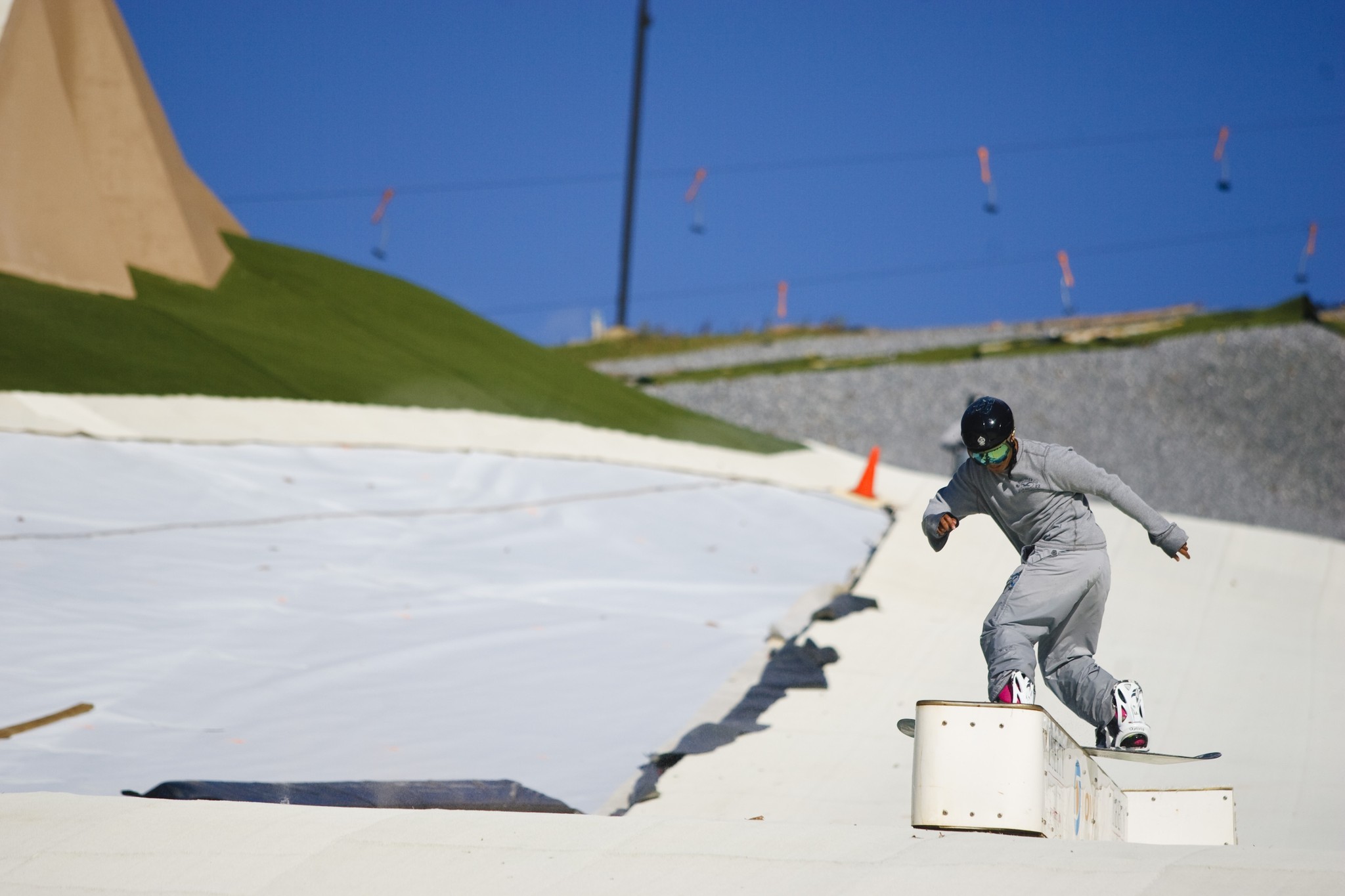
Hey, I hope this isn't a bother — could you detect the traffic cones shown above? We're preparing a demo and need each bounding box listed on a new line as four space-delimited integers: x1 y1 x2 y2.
848 445 881 500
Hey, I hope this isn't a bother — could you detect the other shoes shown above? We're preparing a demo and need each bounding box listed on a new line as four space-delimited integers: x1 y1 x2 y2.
1110 680 1151 752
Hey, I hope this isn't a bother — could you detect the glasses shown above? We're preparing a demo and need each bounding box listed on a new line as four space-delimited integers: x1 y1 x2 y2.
967 433 1012 465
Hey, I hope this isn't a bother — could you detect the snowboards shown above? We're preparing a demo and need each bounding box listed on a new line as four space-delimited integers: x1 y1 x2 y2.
896 714 1222 766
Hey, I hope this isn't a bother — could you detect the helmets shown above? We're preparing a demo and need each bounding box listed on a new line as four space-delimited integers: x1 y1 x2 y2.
960 397 1016 466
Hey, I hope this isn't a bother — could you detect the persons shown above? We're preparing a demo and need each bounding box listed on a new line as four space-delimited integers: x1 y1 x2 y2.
921 396 1192 759
